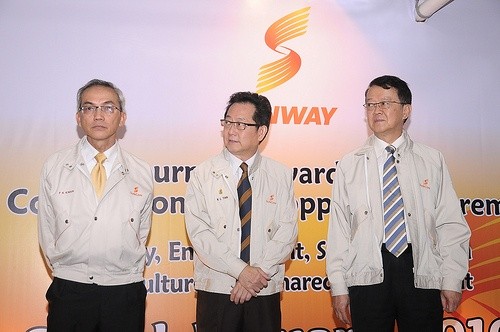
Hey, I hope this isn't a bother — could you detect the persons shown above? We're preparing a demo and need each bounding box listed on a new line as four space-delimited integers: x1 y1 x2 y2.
184 92 298 332
325 75 472 332
38 79 155 332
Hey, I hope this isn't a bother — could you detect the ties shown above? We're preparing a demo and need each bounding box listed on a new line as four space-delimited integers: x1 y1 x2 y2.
383 145 408 258
237 162 252 266
90 153 107 203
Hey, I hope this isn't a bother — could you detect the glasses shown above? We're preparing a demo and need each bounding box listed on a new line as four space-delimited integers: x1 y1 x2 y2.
220 119 257 130
363 102 404 111
77 104 122 114
